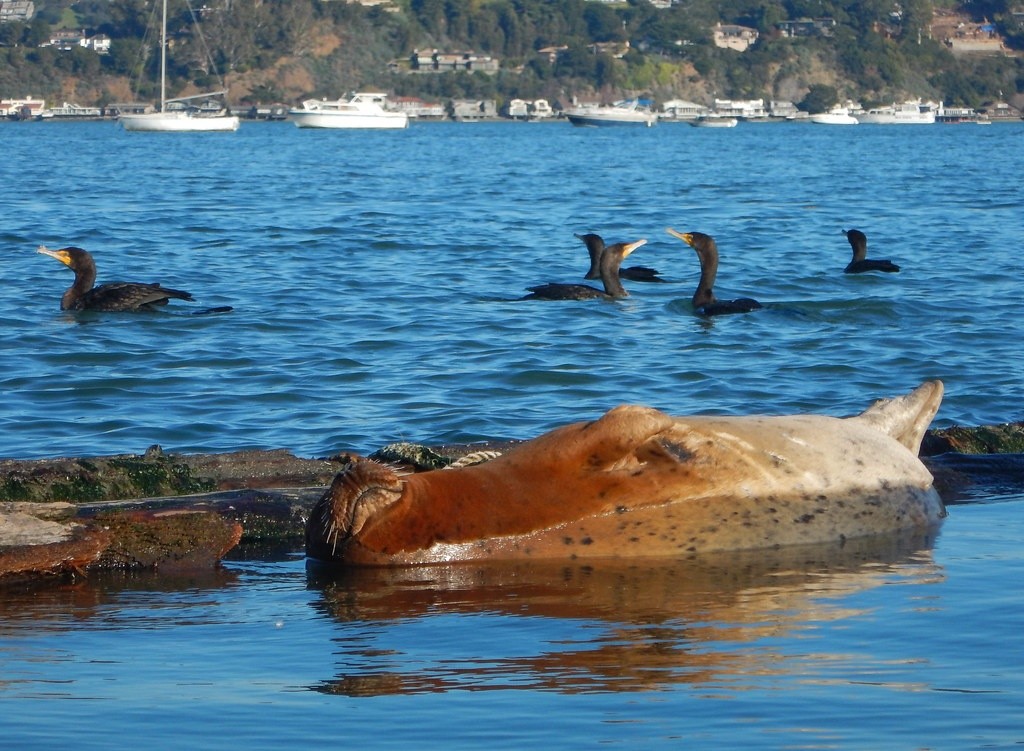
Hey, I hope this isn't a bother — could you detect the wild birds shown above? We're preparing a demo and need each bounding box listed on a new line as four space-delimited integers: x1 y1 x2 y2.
667 228 765 316
519 233 662 302
841 228 903 274
36 245 196 313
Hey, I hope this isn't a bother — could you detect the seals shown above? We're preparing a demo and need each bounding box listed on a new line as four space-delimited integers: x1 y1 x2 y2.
306 380 944 563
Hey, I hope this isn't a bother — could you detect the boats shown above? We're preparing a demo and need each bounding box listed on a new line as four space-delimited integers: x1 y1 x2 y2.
936 101 992 125
1 98 45 118
712 97 771 121
770 101 799 117
289 92 409 128
231 104 289 121
853 100 939 124
39 102 102 121
166 89 229 117
104 103 156 120
661 106 722 124
688 116 738 128
808 106 860 124
562 95 658 128
747 116 771 122
785 116 796 120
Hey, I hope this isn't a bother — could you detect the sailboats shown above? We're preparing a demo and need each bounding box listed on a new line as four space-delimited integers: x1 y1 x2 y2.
117 0 239 132
